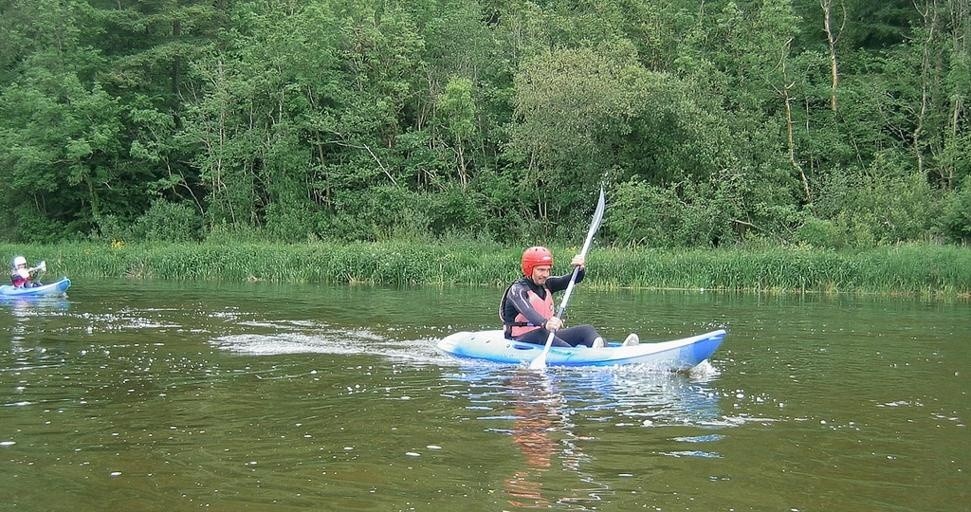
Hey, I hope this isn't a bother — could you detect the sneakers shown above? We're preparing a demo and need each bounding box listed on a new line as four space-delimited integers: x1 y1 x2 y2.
592 337 604 348
622 334 640 347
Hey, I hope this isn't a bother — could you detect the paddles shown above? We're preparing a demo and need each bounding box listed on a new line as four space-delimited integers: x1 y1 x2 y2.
530 186 605 372
18 261 46 279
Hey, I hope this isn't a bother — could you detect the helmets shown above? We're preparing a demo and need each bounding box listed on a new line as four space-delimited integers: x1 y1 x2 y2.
522 246 553 277
15 256 27 269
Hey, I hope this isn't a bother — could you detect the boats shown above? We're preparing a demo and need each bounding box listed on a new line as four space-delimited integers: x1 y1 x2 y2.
436 330 726 369
0 277 71 297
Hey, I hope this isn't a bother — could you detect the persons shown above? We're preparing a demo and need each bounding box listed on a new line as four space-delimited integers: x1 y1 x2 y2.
500 246 640 349
9 256 44 289
